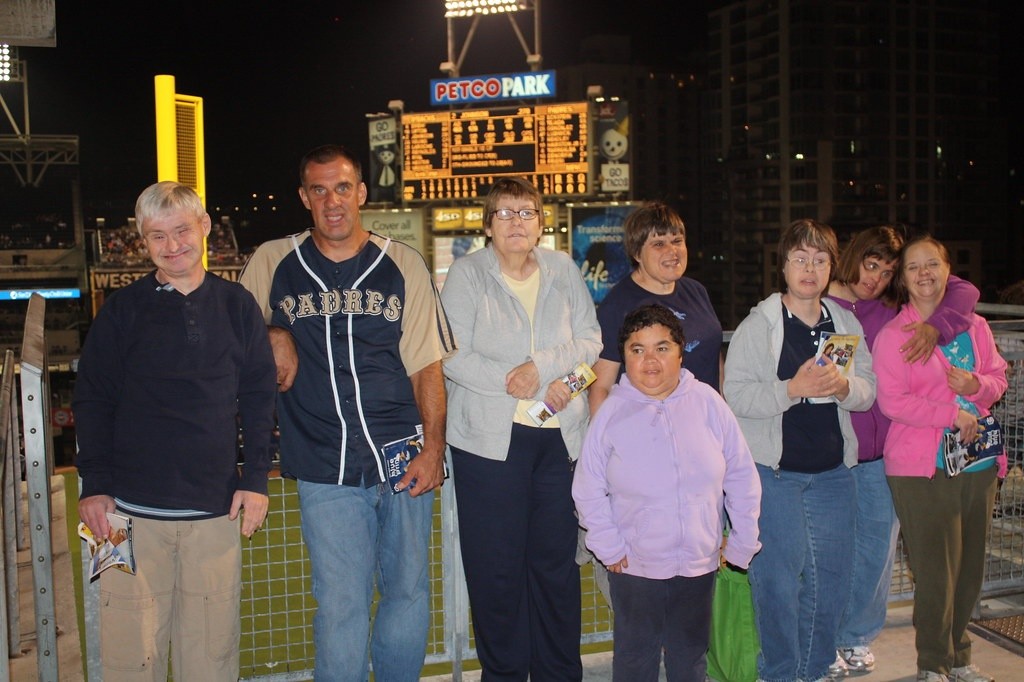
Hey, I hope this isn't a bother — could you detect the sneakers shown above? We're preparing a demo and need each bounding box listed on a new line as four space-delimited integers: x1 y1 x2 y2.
828 650 850 678
916 669 949 681
949 665 995 682
839 645 875 672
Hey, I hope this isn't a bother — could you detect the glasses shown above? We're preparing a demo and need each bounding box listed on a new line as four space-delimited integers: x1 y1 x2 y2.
785 256 833 269
490 208 541 221
863 260 893 281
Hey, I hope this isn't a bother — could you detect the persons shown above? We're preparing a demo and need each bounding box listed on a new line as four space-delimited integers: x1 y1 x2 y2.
70 179 277 682
238 144 458 682
818 225 981 679
871 233 1006 682
568 308 763 682
586 197 724 420
440 176 604 682
725 220 877 682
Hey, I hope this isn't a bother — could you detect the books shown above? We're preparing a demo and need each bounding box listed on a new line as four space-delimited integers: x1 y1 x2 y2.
526 362 599 428
806 331 861 404
76 512 138 582
380 432 449 496
942 412 1004 480
